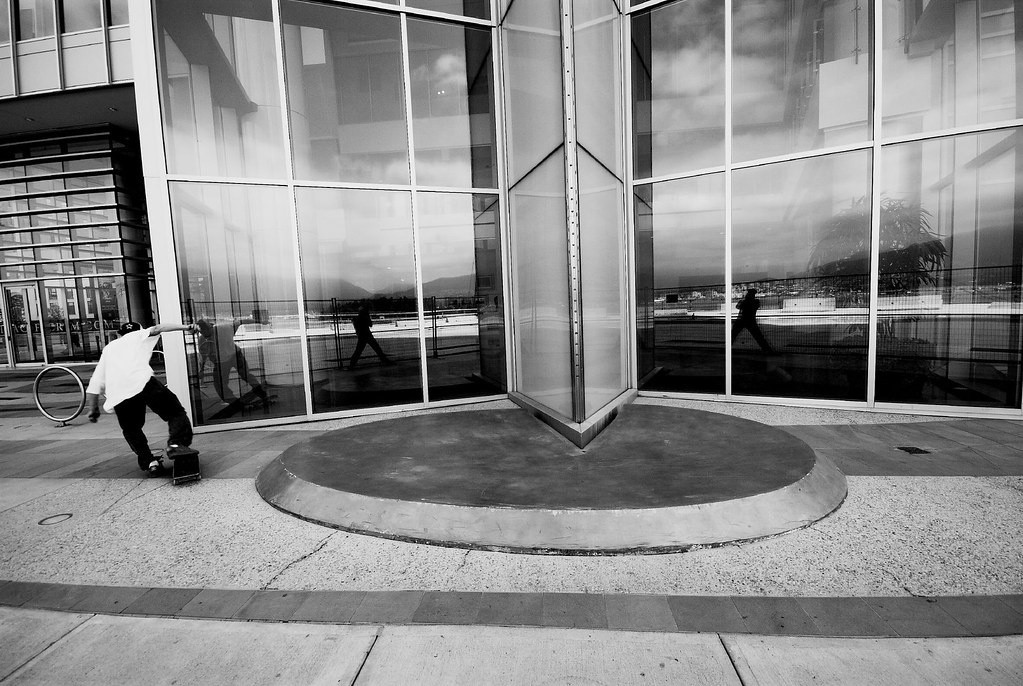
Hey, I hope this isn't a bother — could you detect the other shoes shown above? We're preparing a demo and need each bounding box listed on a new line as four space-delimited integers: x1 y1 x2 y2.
350 362 360 367
167 446 199 459
148 459 164 477
377 353 387 357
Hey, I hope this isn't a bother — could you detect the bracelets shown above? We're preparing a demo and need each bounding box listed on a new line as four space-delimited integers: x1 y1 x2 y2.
89 407 98 411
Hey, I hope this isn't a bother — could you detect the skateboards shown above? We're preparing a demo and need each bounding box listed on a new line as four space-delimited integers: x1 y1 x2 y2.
172 453 201 486
246 394 278 411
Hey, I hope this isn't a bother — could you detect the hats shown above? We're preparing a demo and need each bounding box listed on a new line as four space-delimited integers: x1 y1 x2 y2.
118 321 140 334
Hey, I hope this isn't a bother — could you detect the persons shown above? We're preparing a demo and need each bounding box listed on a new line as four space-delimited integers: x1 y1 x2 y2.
732 289 772 352
86 322 201 476
349 301 396 370
198 319 273 407
